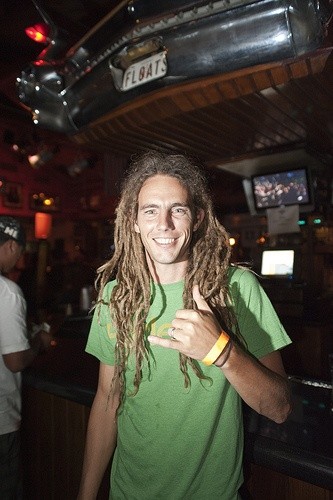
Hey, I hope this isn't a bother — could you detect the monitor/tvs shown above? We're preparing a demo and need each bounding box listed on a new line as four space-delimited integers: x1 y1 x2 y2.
251 167 310 211
260 248 295 278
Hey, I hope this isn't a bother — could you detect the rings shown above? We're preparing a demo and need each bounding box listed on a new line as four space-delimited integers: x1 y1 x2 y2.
171 328 176 341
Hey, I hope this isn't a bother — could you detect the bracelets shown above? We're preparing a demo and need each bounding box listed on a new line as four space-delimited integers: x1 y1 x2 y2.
202 329 233 368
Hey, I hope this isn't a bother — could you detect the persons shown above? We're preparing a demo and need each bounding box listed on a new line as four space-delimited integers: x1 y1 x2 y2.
0 215 53 499
76 151 294 500
253 179 307 207
